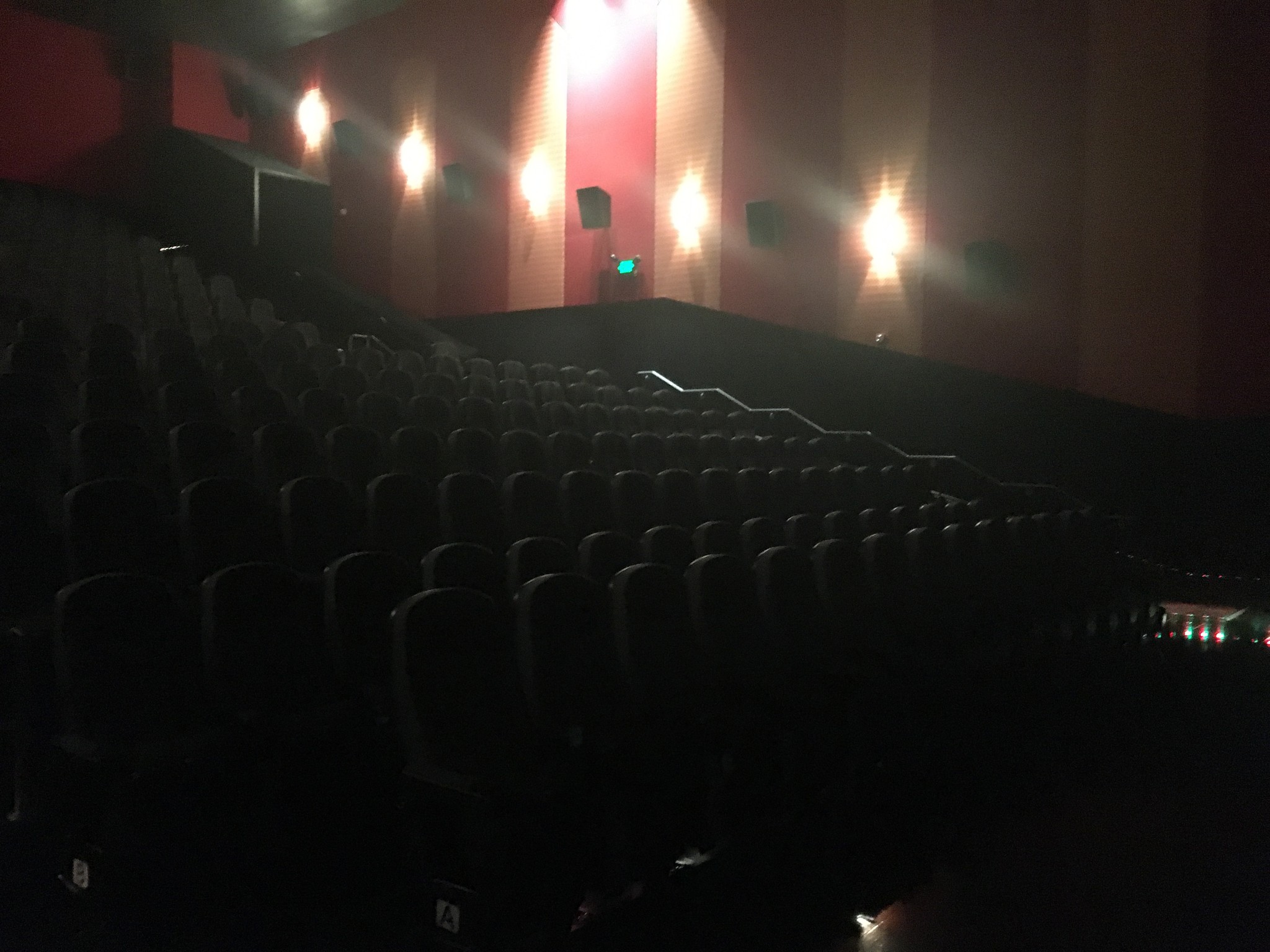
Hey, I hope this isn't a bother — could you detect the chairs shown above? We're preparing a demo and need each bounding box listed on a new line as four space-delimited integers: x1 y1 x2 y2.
0 175 1270 952
